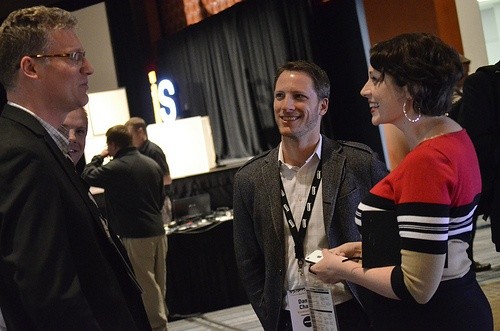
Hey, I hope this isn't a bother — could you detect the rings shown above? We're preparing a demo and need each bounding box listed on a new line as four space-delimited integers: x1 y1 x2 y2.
309 264 313 273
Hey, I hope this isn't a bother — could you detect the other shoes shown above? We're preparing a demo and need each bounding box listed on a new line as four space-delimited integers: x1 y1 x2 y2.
473 262 491 272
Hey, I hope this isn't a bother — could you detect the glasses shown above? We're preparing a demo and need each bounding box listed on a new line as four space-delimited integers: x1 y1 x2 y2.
15 48 88 69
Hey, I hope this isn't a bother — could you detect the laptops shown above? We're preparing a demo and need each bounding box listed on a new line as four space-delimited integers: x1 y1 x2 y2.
169 194 210 228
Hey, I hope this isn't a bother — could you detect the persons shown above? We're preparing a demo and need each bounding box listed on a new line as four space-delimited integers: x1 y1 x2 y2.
307 31 495 331
0 6 177 331
444 52 500 273
231 58 390 330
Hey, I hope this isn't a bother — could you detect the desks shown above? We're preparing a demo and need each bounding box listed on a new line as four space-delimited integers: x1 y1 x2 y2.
164 210 250 324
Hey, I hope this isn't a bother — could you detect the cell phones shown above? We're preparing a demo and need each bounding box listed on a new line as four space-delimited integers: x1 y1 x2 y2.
305 249 349 264
